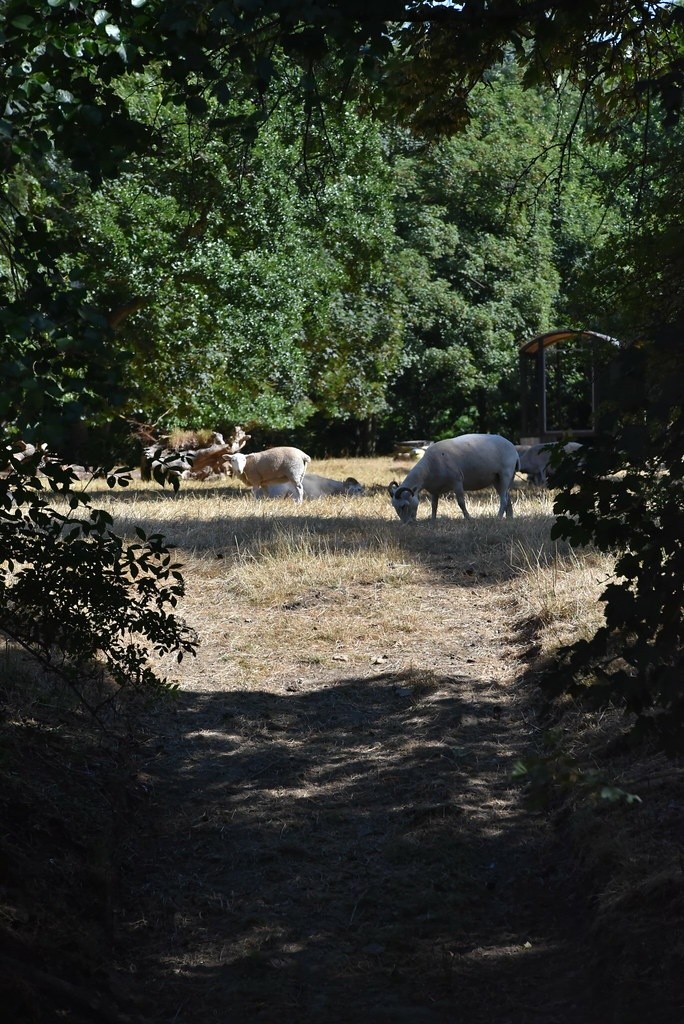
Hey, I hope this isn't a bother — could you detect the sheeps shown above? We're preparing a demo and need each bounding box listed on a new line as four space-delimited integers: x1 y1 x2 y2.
256 474 365 501
387 433 521 523
145 426 253 489
512 442 583 485
222 446 312 503
